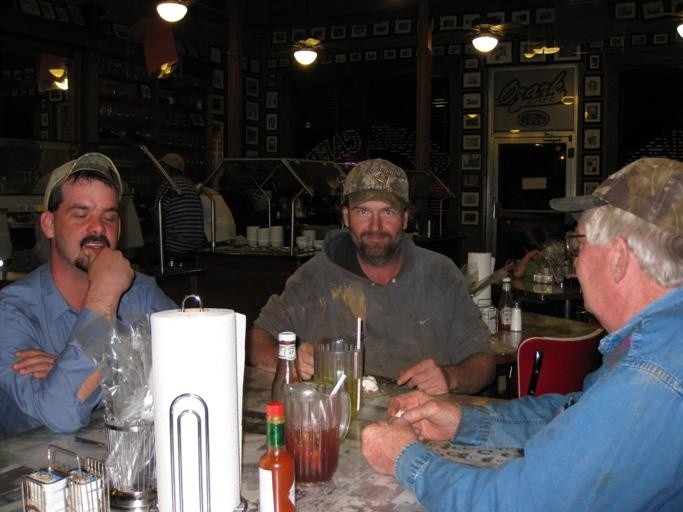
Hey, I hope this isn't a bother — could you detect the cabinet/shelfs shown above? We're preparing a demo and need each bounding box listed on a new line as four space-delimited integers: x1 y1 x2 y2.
201 157 456 331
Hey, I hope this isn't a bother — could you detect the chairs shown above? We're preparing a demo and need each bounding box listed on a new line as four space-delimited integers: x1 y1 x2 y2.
516 327 605 400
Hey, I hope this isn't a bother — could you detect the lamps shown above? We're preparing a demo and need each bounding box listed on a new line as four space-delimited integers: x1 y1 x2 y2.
157 1 188 23
292 41 317 68
472 24 500 55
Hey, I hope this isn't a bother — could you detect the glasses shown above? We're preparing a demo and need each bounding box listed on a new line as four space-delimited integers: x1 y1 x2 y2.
564 230 602 261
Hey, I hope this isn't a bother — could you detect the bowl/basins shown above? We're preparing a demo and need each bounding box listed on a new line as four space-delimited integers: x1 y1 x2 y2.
247 226 283 250
296 231 324 250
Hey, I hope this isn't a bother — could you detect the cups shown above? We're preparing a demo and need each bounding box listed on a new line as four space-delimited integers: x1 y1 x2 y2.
284 381 352 488
315 341 360 426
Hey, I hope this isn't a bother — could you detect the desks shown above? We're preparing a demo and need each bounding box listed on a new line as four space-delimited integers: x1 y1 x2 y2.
475 305 606 401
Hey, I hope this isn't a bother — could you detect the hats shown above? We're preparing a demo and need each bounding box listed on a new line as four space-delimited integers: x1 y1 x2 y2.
339 157 411 211
548 155 683 242
153 153 186 175
43 150 124 213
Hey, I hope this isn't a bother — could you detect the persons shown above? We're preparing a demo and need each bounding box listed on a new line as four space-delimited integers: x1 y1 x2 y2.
500 220 546 279
192 177 236 247
246 158 498 397
360 157 683 512
142 152 205 266
0 151 182 442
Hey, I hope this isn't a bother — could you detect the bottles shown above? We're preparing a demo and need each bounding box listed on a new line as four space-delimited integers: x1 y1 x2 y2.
533 252 579 288
1 64 50 184
499 332 523 351
410 217 439 240
258 404 298 510
102 73 204 188
273 331 300 405
498 276 525 332
